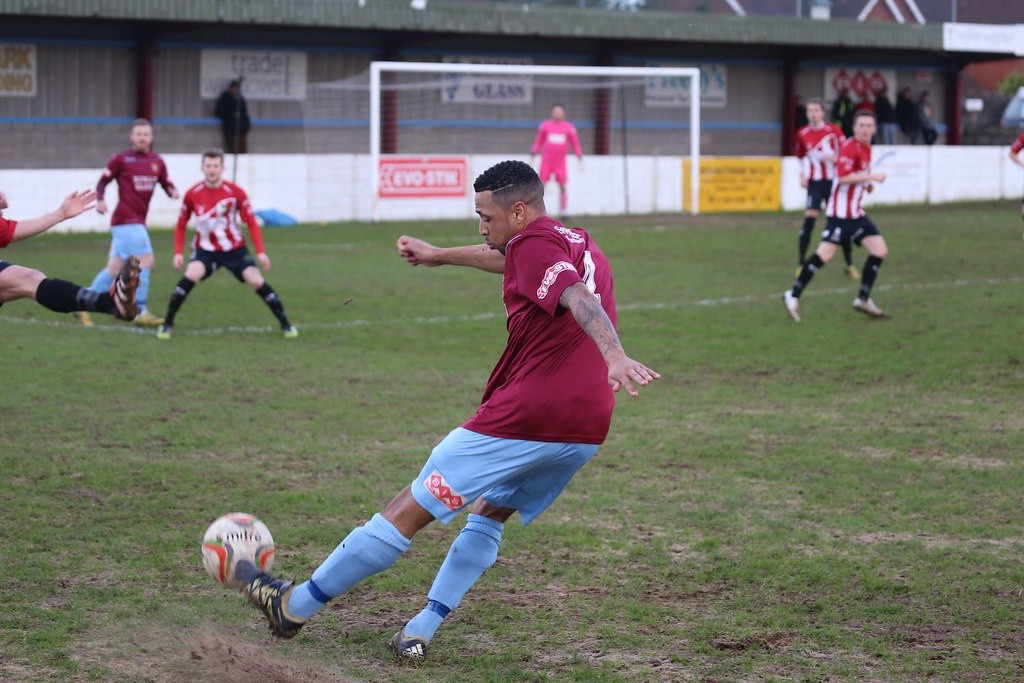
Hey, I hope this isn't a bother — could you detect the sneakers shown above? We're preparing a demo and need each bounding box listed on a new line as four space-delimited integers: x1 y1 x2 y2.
782 290 801 324
110 255 141 322
389 621 427 660
234 560 306 637
854 298 887 317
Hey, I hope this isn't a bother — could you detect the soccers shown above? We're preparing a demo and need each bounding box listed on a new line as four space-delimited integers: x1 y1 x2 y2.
200 513 274 591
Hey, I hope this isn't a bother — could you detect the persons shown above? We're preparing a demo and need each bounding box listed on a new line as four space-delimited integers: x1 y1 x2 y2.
0 188 142 322
73 118 178 327
156 148 299 339
530 104 582 219
1009 132 1024 239
213 79 251 153
829 86 937 144
235 161 662 660
782 110 888 322
794 95 807 130
793 98 860 279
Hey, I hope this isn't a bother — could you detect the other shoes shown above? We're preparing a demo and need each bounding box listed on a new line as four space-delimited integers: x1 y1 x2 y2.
285 327 298 338
796 266 801 278
156 324 171 340
76 311 94 327
846 266 860 280
137 311 164 326
